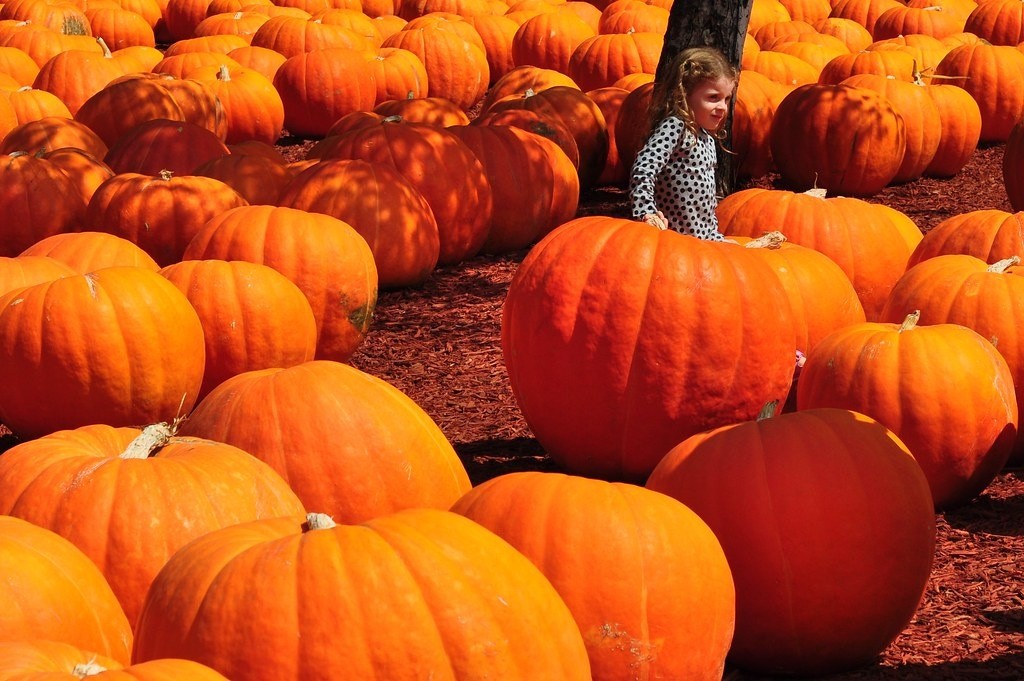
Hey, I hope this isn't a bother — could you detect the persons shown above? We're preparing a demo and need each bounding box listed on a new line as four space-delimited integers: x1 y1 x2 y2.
628 46 743 246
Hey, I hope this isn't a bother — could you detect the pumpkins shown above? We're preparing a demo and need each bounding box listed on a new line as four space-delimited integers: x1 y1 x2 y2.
0 0 1024 681
